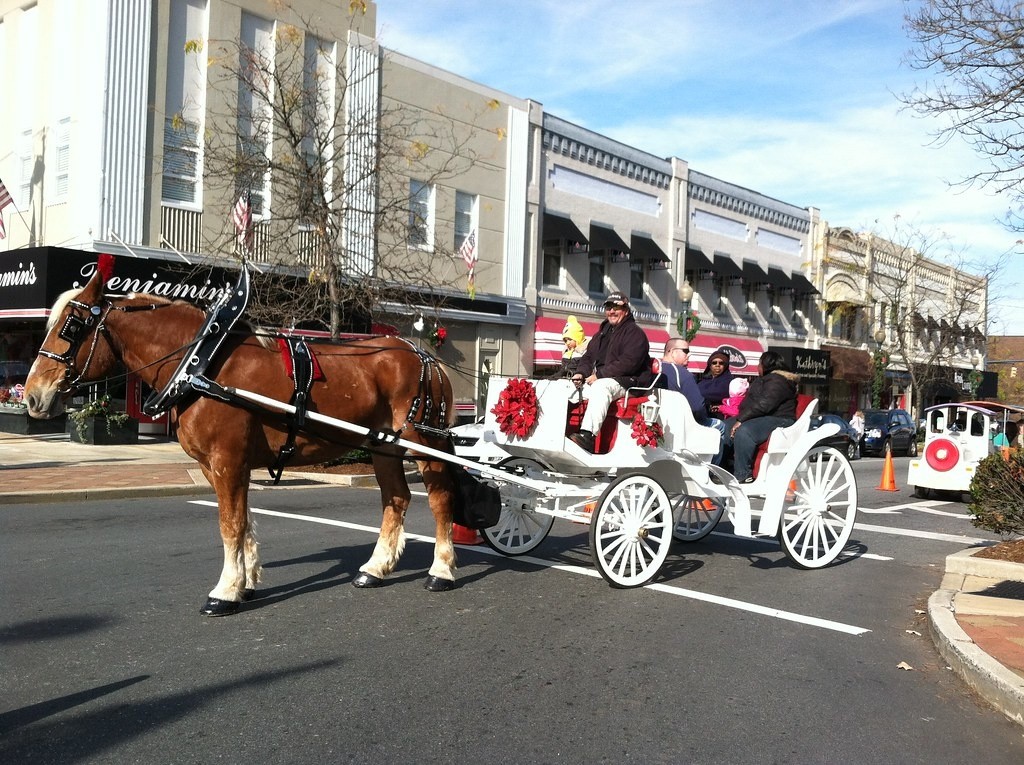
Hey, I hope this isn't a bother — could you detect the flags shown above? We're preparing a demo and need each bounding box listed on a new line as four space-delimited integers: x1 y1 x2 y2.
234 183 253 243
460 230 474 287
0 178 13 239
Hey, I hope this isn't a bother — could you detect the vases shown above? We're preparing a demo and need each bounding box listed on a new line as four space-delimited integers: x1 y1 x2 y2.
68 414 138 445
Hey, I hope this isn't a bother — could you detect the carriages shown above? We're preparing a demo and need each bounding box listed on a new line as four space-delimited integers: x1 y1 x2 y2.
17 263 859 620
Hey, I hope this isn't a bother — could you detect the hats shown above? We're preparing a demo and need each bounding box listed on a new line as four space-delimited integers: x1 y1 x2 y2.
602 292 628 309
562 315 585 358
707 352 729 367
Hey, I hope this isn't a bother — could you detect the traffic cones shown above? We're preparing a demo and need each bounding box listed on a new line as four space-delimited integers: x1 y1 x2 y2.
873 451 900 492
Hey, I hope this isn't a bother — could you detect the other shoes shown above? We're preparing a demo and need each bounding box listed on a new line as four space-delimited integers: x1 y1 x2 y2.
713 475 753 484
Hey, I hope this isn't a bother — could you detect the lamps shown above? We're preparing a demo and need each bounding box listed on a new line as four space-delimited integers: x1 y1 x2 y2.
640 388 661 428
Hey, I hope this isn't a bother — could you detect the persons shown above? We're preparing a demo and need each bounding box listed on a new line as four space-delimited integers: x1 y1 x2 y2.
658 338 726 484
696 351 734 421
849 410 865 459
716 351 799 484
711 378 750 422
954 408 1024 460
550 316 596 379
564 292 653 452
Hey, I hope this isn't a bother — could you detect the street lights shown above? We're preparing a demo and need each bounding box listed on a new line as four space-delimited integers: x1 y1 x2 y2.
970 356 979 401
873 329 886 409
677 279 695 341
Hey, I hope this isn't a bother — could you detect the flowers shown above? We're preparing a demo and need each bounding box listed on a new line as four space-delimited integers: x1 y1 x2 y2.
426 324 447 353
490 377 540 439
630 413 665 449
70 393 130 443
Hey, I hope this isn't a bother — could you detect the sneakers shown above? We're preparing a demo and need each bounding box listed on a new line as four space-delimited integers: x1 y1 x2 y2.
570 430 594 453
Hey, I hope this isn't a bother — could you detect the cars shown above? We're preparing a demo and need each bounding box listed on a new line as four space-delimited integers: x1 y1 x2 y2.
807 414 861 462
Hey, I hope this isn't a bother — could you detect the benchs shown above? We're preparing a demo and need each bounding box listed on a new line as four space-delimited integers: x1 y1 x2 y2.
649 387 721 455
751 393 819 480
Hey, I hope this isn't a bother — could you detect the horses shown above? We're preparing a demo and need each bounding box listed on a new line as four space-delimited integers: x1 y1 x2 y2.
21 272 463 617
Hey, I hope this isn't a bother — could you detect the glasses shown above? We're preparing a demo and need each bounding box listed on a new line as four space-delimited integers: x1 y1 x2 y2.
668 348 688 354
712 361 725 366
605 306 625 311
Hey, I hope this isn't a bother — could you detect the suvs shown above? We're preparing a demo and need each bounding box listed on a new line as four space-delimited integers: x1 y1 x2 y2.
851 407 918 458
